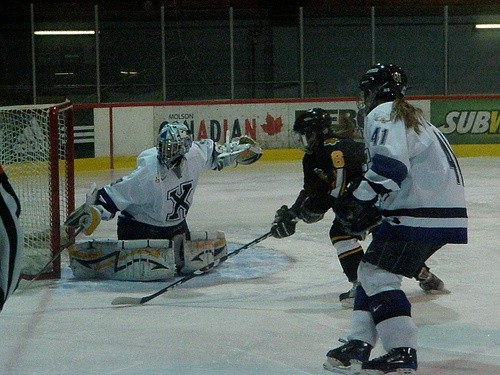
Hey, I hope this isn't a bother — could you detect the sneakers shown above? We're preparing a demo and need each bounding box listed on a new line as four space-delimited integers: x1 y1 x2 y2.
416 267 451 294
358 346 418 375
322 340 374 375
339 282 360 308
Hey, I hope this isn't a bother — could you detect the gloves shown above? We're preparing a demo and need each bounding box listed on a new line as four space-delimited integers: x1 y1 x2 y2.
298 196 326 224
270 205 296 239
334 177 377 224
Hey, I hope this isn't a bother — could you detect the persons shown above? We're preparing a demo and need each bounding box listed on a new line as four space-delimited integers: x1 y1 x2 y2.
270 107 444 301
326 62 468 372
63 119 264 282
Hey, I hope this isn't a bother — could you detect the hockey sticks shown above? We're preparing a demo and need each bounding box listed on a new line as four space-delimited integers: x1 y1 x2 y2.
18 224 86 293
110 214 304 308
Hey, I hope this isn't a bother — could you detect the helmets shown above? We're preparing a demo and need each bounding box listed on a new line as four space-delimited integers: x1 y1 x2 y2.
293 107 332 154
356 62 408 116
156 122 192 169
